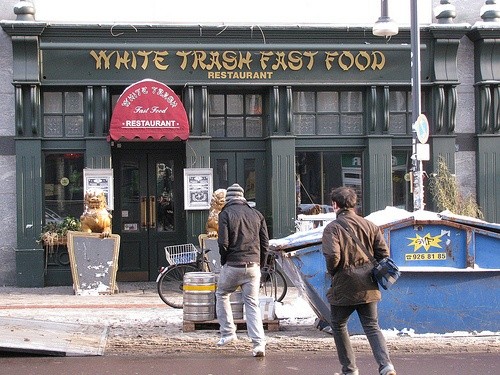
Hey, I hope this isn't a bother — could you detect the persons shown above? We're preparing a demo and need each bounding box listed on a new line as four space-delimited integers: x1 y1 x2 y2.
321 186 396 375
215 182 267 358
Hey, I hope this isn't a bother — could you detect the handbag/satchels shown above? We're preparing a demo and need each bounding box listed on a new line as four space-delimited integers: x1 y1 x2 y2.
373 258 401 290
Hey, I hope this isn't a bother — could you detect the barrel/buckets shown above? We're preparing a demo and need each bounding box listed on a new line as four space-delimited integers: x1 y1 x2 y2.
226 291 244 320
183 272 216 321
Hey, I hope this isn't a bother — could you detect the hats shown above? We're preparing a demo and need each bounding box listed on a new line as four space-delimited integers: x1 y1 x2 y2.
225 184 244 200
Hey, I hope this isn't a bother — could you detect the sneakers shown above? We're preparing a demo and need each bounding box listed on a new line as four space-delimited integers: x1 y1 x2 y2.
254 346 265 357
217 334 237 346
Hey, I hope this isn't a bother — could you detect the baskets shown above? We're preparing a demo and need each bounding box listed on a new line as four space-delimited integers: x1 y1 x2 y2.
165 243 197 266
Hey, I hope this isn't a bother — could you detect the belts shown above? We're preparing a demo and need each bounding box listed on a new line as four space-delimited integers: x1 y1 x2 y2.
228 263 260 268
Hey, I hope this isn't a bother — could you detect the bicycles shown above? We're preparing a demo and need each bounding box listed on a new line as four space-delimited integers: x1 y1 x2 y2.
154 242 289 310
